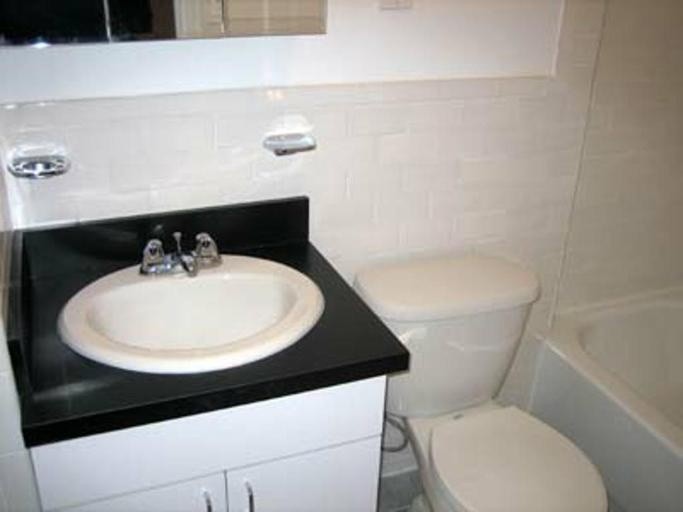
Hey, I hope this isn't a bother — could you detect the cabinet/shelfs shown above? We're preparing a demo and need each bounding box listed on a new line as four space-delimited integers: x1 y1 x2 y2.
26 373 411 512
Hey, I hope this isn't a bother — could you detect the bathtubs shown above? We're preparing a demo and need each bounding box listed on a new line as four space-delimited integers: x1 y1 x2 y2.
532 295 683 512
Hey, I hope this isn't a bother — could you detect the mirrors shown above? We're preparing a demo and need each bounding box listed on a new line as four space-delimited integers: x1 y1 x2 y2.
0 0 329 46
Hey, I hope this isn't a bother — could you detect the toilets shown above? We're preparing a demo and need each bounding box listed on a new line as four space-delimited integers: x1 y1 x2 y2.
352 256 609 512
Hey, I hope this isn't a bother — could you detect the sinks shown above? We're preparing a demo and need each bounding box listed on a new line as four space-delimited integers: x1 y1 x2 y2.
57 254 325 376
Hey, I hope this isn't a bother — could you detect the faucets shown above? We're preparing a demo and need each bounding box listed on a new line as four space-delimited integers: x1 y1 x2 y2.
170 231 200 277
